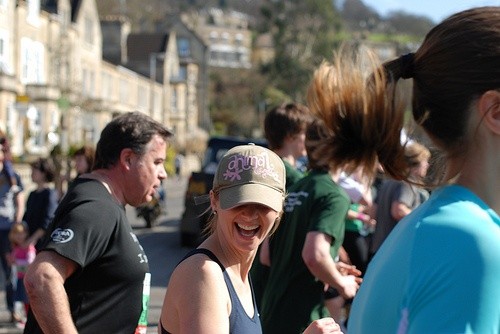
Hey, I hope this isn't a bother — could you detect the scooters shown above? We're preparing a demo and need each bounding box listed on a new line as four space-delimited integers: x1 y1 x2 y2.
134 189 164 227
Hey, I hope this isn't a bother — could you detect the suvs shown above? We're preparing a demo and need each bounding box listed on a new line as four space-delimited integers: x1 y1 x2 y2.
179 135 269 250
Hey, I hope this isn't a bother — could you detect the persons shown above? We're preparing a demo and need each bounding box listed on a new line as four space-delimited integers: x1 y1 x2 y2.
0 136 95 330
22 112 172 334
159 144 344 334
248 101 435 333
306 5 499 333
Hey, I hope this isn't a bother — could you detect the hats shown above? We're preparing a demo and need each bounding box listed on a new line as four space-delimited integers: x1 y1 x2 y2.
212 142 287 213
